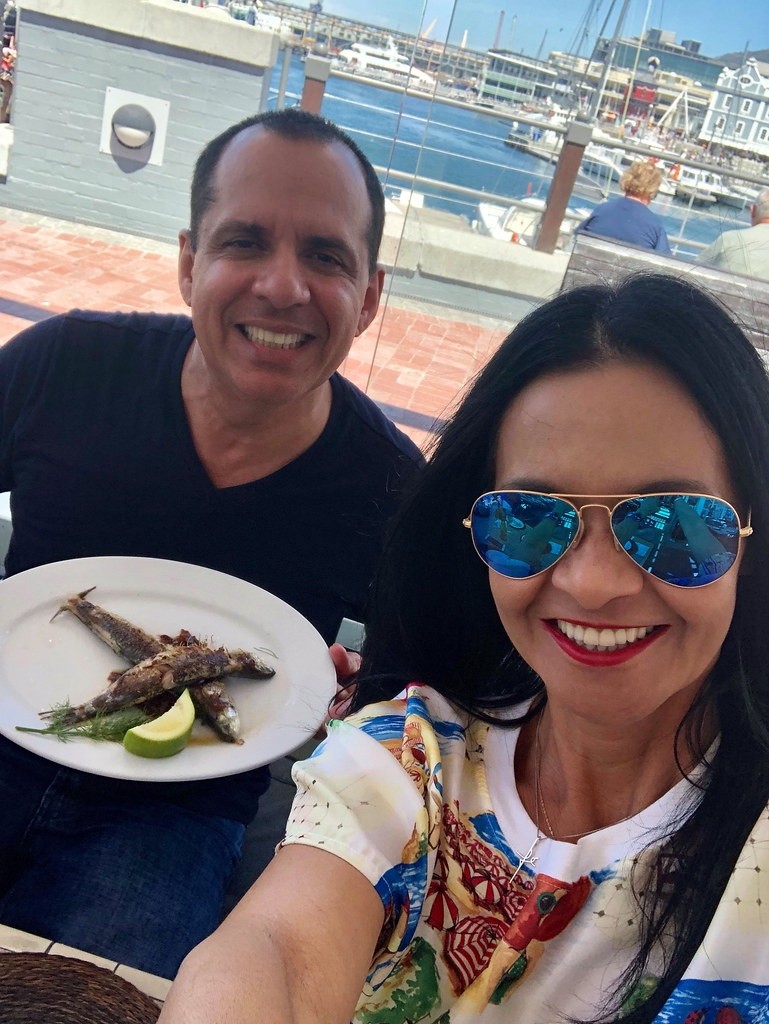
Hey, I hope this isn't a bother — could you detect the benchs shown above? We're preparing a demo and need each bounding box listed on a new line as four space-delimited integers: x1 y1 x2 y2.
560 230 769 354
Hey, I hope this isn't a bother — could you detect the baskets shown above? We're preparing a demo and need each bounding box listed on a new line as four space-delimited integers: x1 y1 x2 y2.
1 951 161 1024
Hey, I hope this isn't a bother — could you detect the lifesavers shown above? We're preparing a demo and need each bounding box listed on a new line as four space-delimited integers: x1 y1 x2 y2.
668 164 680 180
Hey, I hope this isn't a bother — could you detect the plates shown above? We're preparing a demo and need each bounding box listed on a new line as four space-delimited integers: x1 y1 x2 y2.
0 556 337 782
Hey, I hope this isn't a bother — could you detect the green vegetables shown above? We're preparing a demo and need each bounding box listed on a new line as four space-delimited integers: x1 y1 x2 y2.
16 700 158 747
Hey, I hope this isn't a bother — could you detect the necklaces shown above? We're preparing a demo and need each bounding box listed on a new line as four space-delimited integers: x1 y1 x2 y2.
507 694 637 885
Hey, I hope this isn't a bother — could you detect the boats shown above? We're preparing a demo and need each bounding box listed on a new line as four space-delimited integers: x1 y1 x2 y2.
328 32 442 96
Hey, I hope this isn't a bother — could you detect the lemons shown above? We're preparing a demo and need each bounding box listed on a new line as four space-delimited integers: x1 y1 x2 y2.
122 688 196 757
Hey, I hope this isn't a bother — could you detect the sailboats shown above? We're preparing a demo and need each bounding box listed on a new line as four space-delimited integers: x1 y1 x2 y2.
478 0 769 253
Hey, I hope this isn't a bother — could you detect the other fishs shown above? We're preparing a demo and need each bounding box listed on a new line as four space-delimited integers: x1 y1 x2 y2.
37 641 276 731
50 586 241 743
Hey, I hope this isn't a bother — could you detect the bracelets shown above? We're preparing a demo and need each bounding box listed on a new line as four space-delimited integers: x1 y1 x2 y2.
673 496 686 507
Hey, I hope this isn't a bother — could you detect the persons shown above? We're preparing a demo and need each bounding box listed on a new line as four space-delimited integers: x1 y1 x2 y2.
0 107 428 981
573 163 674 256
688 190 769 282
613 495 737 587
486 501 574 581
147 269 769 1024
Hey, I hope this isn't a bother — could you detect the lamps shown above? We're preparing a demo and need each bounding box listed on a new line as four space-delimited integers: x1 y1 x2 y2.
112 104 155 149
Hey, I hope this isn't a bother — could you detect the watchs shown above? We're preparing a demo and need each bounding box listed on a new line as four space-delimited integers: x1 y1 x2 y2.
543 510 562 524
624 510 644 529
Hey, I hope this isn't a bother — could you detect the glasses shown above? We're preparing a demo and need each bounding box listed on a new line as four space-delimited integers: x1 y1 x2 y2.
461 488 756 589
